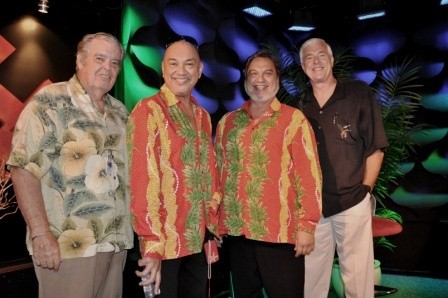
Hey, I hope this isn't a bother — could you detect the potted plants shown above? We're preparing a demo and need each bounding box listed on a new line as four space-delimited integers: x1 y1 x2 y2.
265 39 431 298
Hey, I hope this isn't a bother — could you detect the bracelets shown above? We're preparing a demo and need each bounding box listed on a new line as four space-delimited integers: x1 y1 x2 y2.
32 231 53 241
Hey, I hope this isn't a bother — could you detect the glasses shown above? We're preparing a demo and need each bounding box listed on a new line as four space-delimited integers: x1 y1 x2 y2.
166 35 198 47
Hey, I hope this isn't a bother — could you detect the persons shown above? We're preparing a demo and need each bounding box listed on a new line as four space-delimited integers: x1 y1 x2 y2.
213 49 323 298
6 32 134 298
297 38 388 298
126 35 223 298
